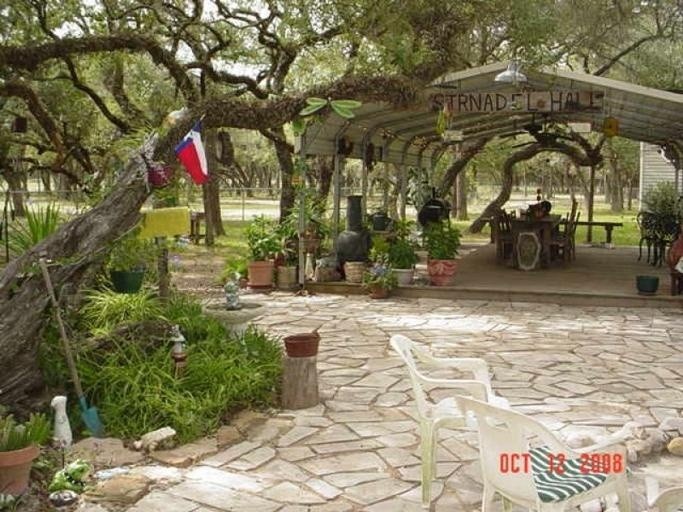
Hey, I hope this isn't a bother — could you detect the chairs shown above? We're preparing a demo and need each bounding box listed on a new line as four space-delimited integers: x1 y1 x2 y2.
479 198 581 266
667 225 683 295
454 394 637 512
635 211 680 267
390 334 513 505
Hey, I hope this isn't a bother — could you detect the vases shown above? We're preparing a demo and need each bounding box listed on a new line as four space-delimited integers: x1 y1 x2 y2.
636 275 659 295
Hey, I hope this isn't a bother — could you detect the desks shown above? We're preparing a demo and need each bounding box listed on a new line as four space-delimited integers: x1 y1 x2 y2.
189 211 206 244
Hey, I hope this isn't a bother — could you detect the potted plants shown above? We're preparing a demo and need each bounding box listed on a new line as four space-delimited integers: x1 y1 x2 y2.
110 230 170 294
245 159 461 301
0 409 52 492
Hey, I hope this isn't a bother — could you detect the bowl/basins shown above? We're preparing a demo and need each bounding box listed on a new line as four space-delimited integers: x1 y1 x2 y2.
637 275 660 292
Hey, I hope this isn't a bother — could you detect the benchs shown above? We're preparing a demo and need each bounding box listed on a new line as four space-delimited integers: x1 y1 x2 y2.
479 217 622 242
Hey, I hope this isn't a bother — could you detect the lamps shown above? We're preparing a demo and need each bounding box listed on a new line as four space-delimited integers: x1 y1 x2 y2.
604 87 618 134
494 57 527 83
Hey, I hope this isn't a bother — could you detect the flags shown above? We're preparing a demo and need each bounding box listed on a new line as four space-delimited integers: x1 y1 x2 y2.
173 115 208 185
